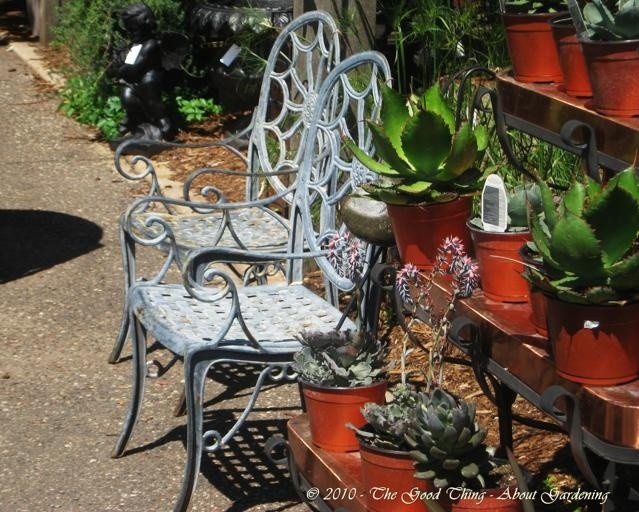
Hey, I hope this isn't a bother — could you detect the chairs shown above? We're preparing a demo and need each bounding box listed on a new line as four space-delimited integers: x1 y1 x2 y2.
110 50 393 512
110 10 340 363
